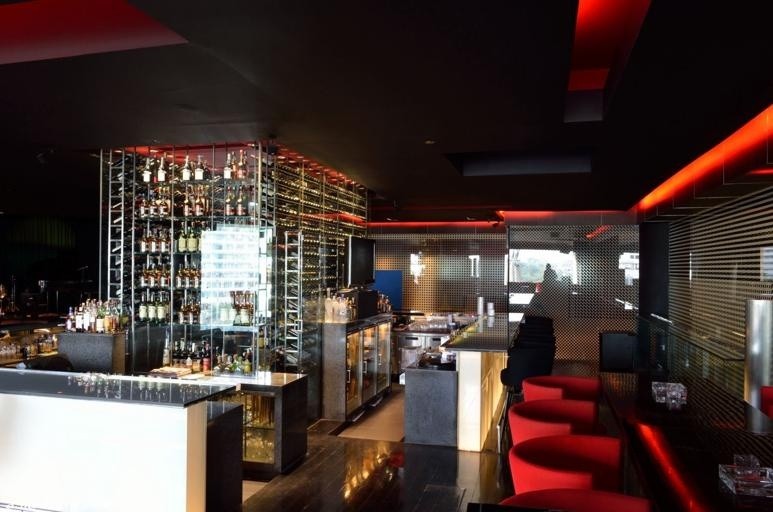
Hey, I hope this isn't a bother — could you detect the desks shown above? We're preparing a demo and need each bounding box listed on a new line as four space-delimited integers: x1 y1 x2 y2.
601 372 773 512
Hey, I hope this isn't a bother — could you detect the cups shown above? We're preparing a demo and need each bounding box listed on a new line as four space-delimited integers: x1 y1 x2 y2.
487 303 495 316
733 454 760 481
477 297 483 315
487 317 495 327
477 315 484 333
666 391 681 409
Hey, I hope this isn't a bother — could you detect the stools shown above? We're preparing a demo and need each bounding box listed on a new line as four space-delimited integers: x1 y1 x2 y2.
498 316 557 451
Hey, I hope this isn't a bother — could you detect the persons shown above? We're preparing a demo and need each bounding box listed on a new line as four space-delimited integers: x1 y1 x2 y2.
543 263 558 281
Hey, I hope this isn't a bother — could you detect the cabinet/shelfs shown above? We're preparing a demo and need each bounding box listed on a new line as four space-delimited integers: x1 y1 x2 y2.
102 140 368 371
323 316 448 421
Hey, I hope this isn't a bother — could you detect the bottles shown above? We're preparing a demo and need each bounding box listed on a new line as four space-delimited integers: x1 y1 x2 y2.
377 291 391 313
111 146 366 325
275 326 319 372
63 297 128 333
159 329 270 374
0 330 58 358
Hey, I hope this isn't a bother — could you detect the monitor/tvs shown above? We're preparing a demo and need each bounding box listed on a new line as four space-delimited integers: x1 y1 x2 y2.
344 234 376 289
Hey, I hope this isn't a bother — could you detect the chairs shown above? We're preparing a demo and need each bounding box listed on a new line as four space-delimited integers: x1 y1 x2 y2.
498 487 651 512
759 385 773 418
523 377 600 402
509 436 624 495
508 398 596 447
